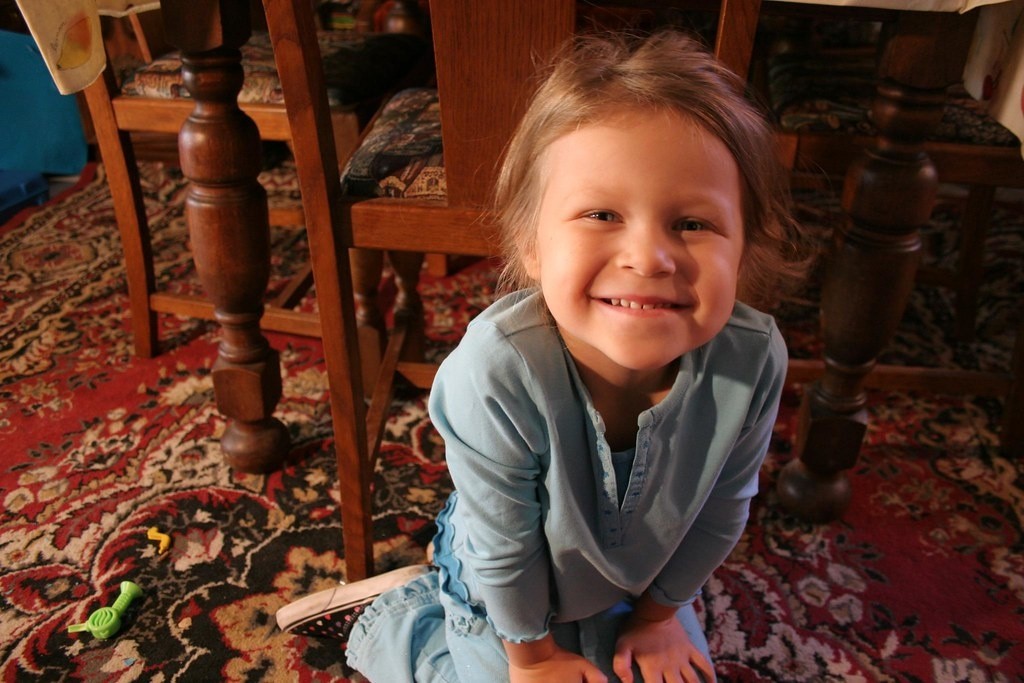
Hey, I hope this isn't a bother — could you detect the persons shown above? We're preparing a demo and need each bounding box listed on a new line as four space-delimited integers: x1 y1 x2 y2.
275 32 791 683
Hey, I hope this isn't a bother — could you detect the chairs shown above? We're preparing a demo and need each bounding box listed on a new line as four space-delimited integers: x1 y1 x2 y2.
16 1 1024 581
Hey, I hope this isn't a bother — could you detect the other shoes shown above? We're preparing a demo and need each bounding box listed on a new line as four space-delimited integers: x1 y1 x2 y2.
274 565 429 650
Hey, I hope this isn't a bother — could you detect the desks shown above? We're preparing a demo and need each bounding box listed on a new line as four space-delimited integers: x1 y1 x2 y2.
181 2 1002 518
